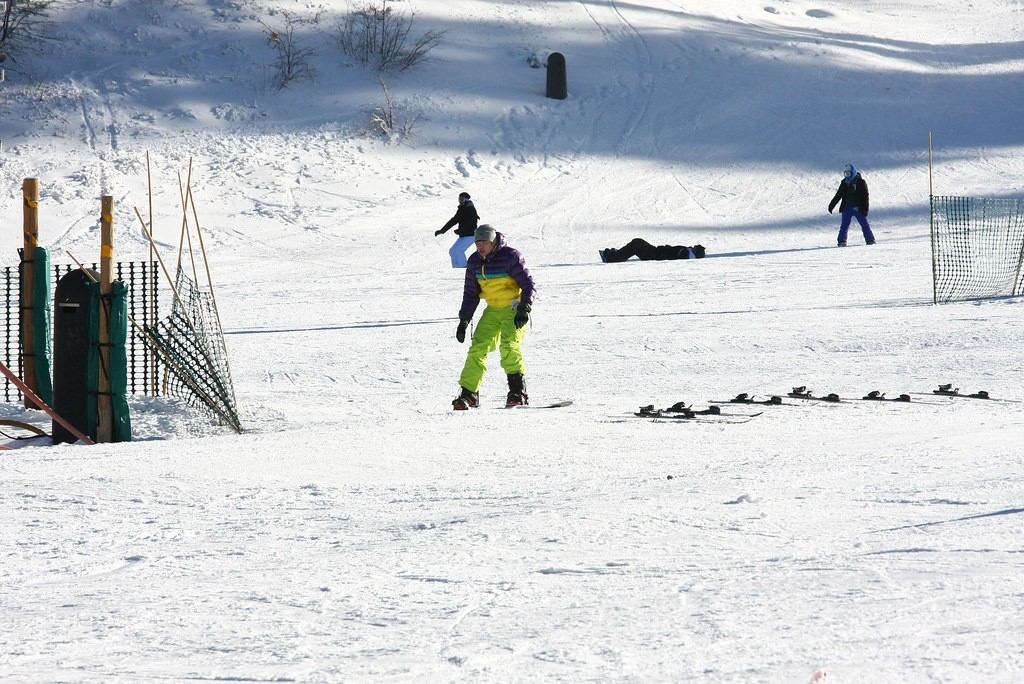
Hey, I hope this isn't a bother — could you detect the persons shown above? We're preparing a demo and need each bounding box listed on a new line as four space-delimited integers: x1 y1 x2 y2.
452 223 537 407
434 191 480 269
829 163 876 246
604 237 705 264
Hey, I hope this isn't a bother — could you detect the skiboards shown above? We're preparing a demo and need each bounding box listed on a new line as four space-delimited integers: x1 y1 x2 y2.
413 399 573 410
599 380 1022 426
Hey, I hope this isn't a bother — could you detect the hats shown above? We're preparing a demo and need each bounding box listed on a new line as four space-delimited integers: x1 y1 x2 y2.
458 192 472 198
474 224 497 245
843 163 854 171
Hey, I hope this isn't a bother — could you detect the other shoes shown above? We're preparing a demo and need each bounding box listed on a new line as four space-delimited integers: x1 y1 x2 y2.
454 395 480 410
505 393 528 407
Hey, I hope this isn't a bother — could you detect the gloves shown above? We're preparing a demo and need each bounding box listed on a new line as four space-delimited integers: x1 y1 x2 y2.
457 312 470 343
513 302 531 328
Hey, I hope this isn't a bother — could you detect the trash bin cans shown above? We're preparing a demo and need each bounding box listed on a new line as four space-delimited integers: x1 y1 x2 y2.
546 53 569 100
52 268 102 445
